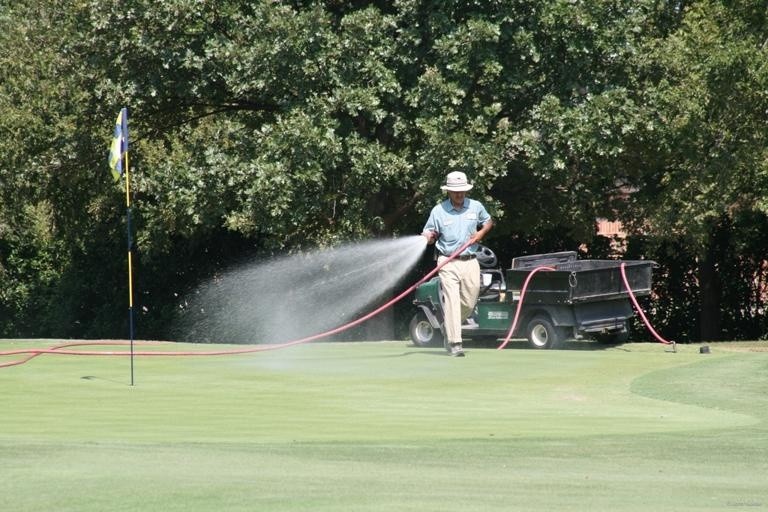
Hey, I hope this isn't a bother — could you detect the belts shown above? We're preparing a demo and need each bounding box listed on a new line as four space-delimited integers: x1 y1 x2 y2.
440 254 477 261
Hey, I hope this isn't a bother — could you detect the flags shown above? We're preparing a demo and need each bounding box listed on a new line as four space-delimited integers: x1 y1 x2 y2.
105 108 128 183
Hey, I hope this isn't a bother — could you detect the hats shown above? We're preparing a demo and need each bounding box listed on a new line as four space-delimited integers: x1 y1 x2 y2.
440 171 474 192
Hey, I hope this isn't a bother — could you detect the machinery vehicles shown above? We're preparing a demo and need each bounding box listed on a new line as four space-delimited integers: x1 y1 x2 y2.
409 251 657 350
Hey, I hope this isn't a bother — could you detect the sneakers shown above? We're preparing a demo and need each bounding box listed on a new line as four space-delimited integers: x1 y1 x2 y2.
440 322 464 357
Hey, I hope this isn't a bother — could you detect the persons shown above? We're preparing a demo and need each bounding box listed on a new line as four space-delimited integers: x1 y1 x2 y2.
418 171 494 359
